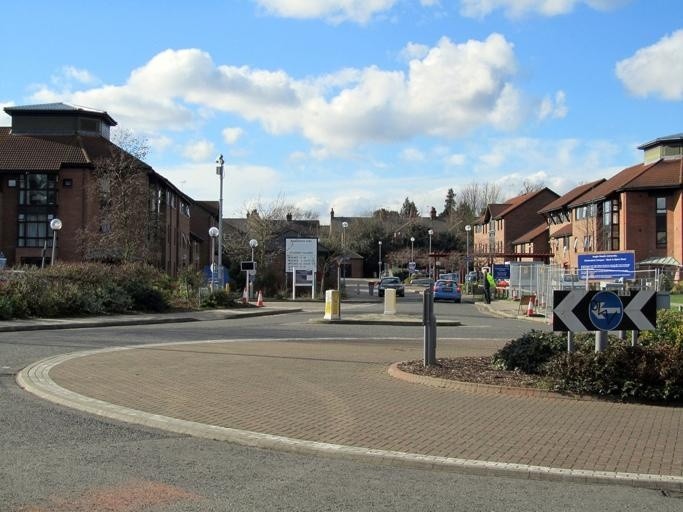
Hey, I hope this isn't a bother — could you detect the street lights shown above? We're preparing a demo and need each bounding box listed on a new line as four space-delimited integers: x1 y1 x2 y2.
49 218 64 267
409 236 415 262
248 238 258 300
341 221 348 295
214 152 224 292
427 229 433 277
464 225 471 282
378 240 383 279
207 227 219 292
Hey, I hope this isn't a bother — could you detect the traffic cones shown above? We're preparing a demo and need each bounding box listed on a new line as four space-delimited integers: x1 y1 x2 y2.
525 296 536 317
240 289 264 308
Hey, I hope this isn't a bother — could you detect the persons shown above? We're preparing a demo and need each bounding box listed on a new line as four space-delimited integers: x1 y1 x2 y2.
483 268 491 303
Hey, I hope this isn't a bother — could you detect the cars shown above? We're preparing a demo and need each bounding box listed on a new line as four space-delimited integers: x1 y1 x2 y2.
378 276 405 296
422 269 484 304
